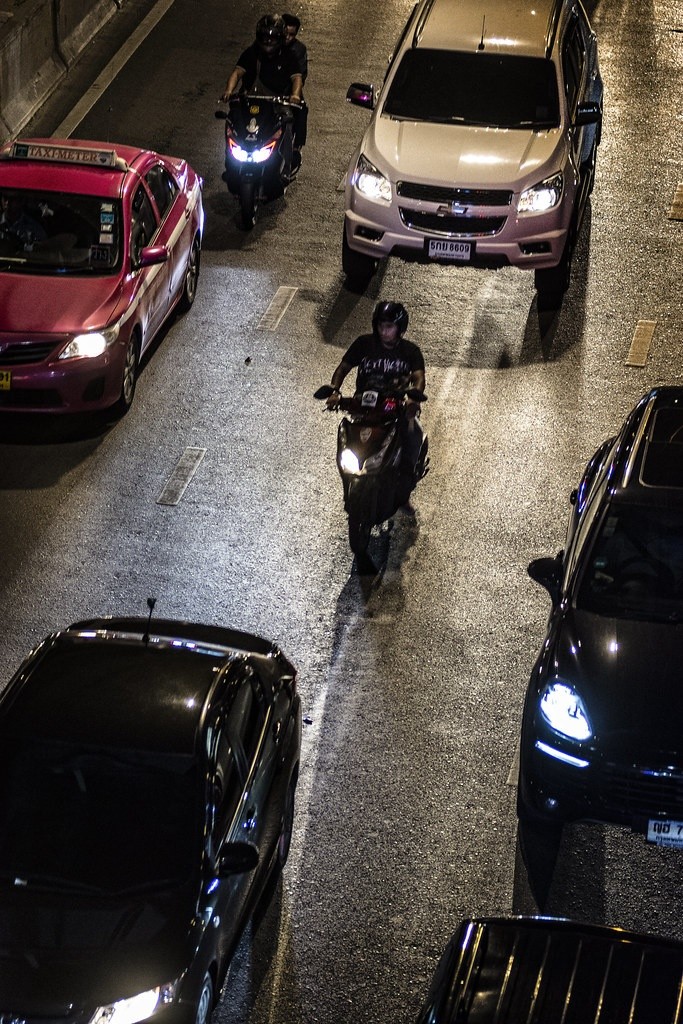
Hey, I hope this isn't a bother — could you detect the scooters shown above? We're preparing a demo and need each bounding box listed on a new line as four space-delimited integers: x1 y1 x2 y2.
215 83 309 232
314 383 425 558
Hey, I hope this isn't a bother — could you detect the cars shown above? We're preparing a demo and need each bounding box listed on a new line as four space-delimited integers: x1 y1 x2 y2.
0 138 205 415
1 613 303 1024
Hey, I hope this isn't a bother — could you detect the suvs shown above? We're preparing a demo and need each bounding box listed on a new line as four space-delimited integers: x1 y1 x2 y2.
516 385 682 849
342 0 605 294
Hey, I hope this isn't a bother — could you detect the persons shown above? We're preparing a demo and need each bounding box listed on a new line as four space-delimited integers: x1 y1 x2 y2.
222 11 309 180
325 300 427 519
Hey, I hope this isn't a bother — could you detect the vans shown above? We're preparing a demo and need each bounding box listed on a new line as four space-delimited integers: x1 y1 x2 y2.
418 912 683 1023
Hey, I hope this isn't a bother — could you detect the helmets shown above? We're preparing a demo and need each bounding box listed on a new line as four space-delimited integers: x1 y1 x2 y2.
372 301 408 335
256 13 288 45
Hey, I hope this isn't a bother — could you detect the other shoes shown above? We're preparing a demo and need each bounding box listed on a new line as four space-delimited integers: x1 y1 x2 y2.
222 171 228 181
398 502 416 519
282 168 291 183
